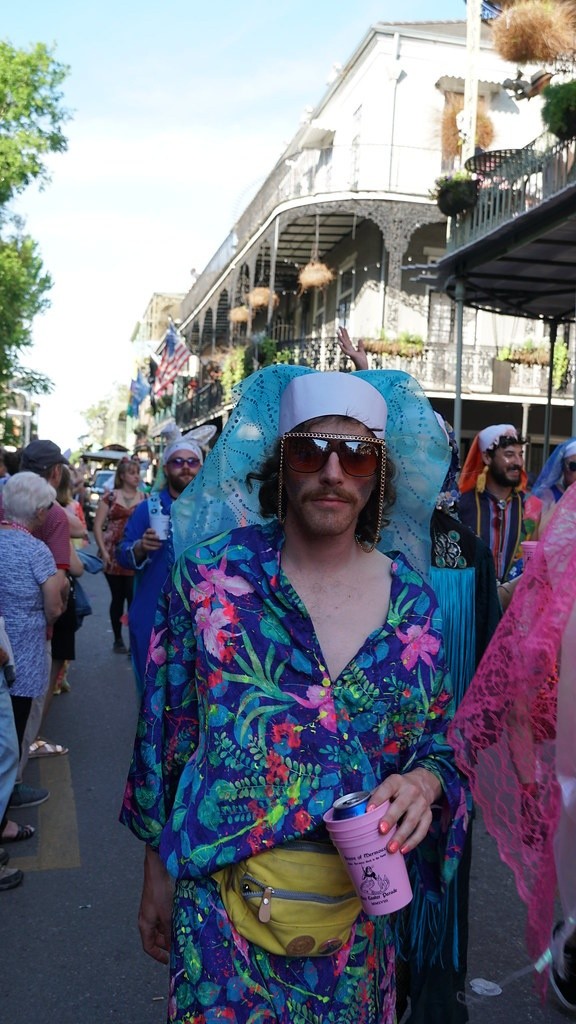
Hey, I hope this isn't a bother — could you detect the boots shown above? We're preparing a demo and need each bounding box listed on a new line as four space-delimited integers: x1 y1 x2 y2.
53 663 71 693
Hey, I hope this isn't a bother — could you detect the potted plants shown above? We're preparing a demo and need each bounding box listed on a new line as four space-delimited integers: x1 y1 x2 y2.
489 343 553 396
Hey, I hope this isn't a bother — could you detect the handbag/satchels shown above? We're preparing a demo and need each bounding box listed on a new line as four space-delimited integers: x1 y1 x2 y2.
96 492 114 558
68 575 92 617
211 841 364 957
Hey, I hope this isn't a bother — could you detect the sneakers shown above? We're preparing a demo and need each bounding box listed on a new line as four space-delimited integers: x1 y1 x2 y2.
0 848 9 863
0 864 23 889
548 920 576 1012
8 784 50 808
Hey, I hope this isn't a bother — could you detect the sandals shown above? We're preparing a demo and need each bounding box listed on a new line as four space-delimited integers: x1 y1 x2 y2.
28 741 68 757
0 821 35 842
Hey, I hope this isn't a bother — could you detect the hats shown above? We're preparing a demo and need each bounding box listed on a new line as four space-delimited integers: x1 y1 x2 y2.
278 371 387 439
23 440 70 469
477 424 518 453
394 405 450 447
161 423 217 465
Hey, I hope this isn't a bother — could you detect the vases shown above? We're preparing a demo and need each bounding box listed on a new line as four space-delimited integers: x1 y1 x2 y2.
435 182 475 217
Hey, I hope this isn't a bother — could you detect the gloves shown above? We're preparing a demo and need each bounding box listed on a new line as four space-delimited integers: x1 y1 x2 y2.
521 783 546 850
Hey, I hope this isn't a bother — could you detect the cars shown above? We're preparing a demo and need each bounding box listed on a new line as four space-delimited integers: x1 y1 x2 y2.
82 470 116 528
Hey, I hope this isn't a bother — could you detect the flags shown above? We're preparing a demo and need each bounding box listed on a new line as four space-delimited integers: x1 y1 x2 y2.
126 325 192 418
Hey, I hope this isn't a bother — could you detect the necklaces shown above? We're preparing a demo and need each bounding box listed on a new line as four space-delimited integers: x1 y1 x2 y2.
2 521 34 537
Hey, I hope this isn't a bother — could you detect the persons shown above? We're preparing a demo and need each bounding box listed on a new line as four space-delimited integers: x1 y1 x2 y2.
0 439 105 892
118 362 477 1024
454 424 541 577
336 325 368 370
529 439 576 501
94 457 151 653
113 441 203 688
447 472 575 1024
431 411 508 766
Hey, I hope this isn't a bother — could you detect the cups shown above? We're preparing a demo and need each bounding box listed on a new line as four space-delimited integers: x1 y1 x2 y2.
150 515 170 540
323 797 413 916
521 541 538 569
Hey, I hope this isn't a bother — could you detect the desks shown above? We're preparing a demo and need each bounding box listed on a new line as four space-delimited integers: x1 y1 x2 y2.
465 146 550 217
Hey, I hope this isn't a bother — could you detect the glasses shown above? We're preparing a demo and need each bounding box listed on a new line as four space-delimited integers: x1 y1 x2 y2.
392 437 448 460
565 462 576 471
167 457 200 468
281 432 384 477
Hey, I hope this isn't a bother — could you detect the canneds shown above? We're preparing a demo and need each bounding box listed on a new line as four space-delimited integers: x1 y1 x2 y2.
332 790 373 821
508 557 524 580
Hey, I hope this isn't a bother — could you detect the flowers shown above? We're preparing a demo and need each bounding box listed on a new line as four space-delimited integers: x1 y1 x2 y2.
427 168 476 199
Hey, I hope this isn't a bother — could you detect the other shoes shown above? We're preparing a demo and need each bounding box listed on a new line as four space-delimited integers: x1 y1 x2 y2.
114 642 126 652
128 648 132 656
